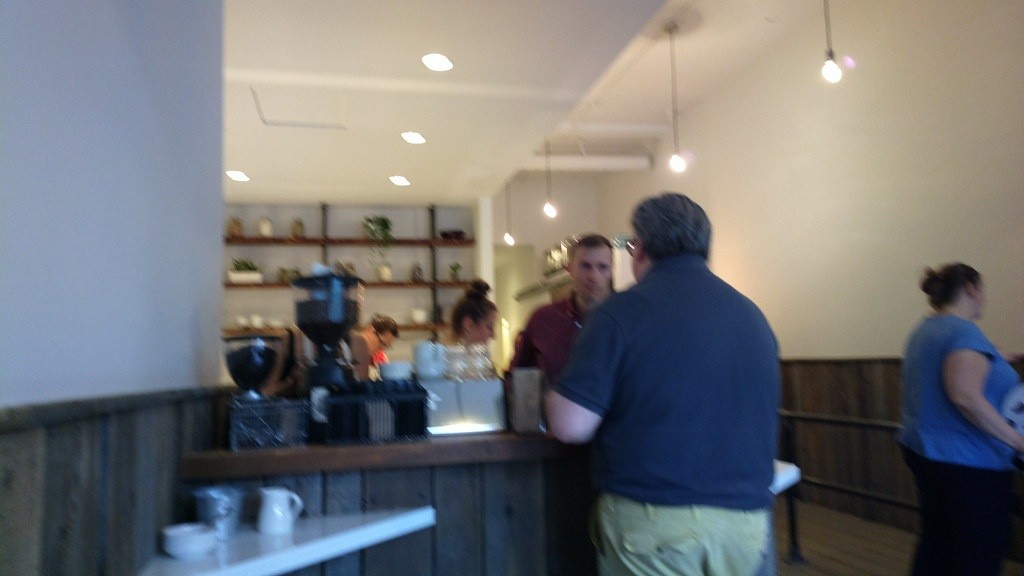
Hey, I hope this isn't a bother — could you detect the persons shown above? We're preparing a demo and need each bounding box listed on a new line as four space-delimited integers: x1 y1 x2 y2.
543 192 785 576
268 273 399 396
897 261 1024 575
451 280 497 349
518 232 616 395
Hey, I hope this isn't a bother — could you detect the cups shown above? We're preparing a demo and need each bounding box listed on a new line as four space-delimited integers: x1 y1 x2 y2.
192 482 242 542
411 341 447 381
443 344 495 381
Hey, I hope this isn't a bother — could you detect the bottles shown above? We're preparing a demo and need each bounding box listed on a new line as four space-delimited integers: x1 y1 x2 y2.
411 262 422 281
229 216 243 238
292 217 304 237
258 215 273 237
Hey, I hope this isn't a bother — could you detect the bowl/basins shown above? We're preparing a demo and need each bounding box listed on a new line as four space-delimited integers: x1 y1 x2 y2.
379 360 412 380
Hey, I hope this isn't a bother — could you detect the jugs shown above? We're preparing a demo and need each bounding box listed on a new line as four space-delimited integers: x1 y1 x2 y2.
258 487 305 535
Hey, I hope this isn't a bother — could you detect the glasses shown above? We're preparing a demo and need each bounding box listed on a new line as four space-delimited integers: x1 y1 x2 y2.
624 238 639 257
390 328 400 339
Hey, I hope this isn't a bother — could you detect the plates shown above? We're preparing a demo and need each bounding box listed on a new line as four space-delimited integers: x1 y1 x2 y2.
162 522 219 560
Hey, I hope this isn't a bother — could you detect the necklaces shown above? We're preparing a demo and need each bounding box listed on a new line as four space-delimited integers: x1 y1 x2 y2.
571 316 584 329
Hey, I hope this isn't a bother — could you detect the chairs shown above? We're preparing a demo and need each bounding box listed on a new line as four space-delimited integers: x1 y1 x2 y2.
772 460 810 567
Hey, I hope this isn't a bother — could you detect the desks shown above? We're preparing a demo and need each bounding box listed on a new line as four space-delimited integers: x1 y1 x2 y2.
139 504 437 576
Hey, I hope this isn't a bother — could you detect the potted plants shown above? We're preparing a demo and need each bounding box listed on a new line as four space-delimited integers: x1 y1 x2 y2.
226 257 265 284
448 262 463 282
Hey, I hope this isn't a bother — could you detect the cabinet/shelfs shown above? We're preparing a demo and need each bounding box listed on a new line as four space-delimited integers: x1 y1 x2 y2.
221 204 477 341
